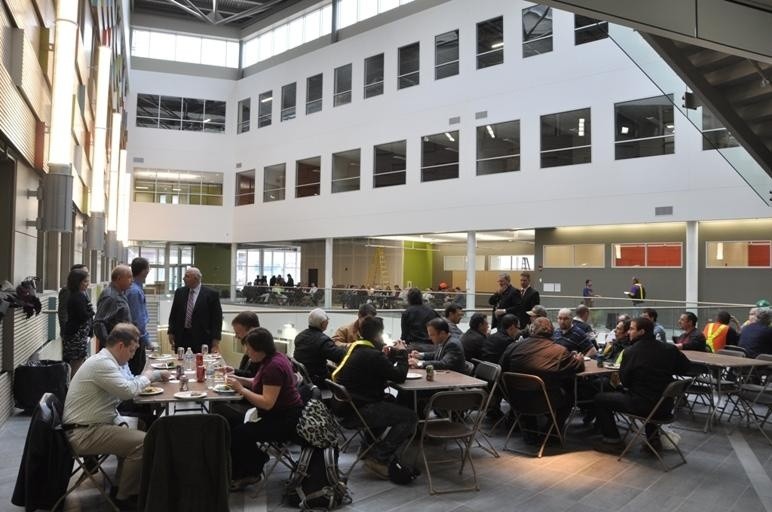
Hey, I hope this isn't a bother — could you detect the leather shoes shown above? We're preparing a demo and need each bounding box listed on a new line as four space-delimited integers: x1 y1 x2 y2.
109 486 138 511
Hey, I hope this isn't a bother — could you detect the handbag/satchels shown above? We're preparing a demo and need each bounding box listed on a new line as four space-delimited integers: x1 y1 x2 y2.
296 398 339 448
17 276 40 298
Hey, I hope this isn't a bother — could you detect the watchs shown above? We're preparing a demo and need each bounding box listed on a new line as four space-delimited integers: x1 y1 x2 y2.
416 360 422 368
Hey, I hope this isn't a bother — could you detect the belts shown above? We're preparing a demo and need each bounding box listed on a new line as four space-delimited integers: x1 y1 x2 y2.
62 423 88 430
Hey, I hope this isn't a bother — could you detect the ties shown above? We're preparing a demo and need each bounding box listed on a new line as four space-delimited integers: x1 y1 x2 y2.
185 291 194 328
521 290 524 295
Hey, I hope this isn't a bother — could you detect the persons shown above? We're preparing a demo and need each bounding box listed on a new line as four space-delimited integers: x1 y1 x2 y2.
737 354 740 358
59 269 96 382
693 297 772 358
61 322 171 509
459 271 695 445
58 263 95 367
224 327 304 491
214 267 465 480
167 267 223 353
92 264 134 354
121 258 160 378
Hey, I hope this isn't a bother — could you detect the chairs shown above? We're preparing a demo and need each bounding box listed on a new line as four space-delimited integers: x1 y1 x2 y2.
244 285 325 306
34 390 235 512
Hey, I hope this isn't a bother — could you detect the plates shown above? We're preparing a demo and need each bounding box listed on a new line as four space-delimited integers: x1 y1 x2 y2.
151 363 176 370
148 354 171 359
405 372 423 379
605 363 620 370
139 387 164 396
212 386 235 394
173 391 208 400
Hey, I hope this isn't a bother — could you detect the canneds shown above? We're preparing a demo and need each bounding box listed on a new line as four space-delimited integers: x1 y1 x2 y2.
195 353 204 367
179 374 188 391
202 345 208 354
177 365 184 380
197 366 205 382
426 365 433 381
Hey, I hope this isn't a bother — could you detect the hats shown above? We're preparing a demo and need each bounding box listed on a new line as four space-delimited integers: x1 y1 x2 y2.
526 305 547 316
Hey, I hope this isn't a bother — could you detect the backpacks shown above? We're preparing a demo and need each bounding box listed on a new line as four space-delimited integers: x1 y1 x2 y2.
280 446 352 512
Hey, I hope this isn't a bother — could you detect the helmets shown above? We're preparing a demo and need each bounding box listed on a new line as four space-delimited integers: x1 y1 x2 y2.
660 430 681 450
390 459 421 484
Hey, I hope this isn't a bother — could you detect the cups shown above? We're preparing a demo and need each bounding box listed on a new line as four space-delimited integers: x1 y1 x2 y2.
200 344 209 355
179 375 190 392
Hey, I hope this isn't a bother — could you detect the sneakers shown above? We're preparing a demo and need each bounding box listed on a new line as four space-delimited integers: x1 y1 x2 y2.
362 458 390 479
230 475 260 491
593 438 625 454
252 451 269 473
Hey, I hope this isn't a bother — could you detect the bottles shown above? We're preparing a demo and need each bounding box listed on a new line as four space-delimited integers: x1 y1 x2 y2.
597 348 605 368
185 347 193 371
205 363 214 390
